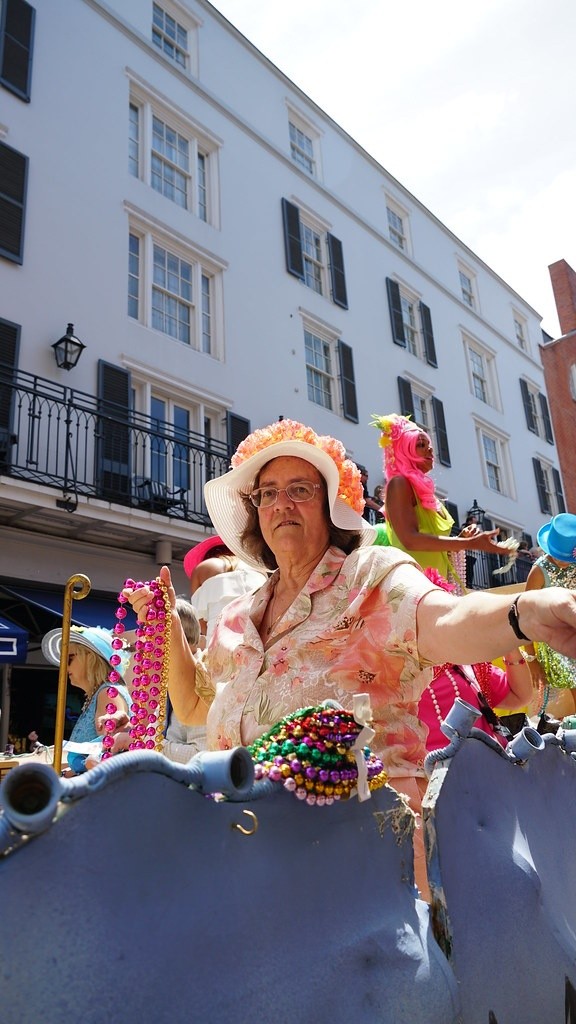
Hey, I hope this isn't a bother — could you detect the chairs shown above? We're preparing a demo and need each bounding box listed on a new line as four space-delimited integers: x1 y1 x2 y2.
131 474 189 523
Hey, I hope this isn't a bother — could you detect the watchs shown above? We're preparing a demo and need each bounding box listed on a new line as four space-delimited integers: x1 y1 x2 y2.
525 654 537 663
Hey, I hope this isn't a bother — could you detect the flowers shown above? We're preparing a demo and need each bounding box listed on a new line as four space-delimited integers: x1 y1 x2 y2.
68 620 134 665
371 410 412 454
228 418 366 519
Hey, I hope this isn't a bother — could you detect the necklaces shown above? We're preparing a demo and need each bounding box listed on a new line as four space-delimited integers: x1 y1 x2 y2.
425 664 461 724
265 581 294 639
82 681 105 714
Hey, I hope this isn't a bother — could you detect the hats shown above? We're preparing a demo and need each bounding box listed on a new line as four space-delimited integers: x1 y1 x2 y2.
537 512 576 563
203 418 379 573
183 535 225 580
41 626 132 686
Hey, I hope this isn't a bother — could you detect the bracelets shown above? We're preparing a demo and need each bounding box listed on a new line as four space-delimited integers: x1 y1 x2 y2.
504 659 526 666
507 593 533 641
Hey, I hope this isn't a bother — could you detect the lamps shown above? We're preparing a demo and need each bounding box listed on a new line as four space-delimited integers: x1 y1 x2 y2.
48 323 86 370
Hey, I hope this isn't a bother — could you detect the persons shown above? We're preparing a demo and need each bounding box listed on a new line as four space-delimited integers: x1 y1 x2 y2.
517 541 543 583
461 515 479 588
371 484 385 524
121 418 574 899
360 470 383 523
40 626 137 777
367 413 514 596
183 535 268 647
419 567 534 750
525 512 576 722
98 598 214 763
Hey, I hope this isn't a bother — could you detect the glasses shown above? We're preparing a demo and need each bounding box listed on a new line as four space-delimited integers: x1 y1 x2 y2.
247 480 322 508
68 651 91 666
